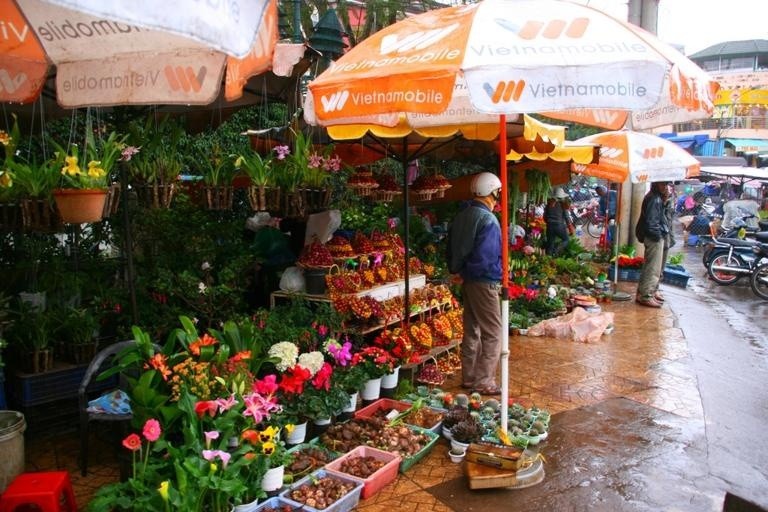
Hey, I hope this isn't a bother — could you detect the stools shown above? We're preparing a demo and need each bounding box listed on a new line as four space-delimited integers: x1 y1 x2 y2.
2 472 77 512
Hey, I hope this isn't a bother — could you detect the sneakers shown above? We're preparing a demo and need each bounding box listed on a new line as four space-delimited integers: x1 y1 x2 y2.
636 291 664 307
462 382 501 395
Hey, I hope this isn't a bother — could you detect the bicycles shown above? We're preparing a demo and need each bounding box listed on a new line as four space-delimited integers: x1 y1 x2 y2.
566 197 604 238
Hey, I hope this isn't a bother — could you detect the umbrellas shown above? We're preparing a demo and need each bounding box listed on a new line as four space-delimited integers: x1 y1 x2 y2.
567 127 702 291
700 165 768 199
0 0 283 110
300 0 724 443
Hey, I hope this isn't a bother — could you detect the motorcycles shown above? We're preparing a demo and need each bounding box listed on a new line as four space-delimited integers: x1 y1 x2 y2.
701 215 766 299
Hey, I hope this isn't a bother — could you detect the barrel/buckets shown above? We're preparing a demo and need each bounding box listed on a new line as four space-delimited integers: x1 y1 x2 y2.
1 410 27 494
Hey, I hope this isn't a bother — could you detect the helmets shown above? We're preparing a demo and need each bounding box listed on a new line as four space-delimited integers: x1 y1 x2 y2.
469 172 502 197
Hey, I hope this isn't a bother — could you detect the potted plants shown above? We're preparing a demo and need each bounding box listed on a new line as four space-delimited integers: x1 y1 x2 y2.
12 302 60 374
62 310 100 366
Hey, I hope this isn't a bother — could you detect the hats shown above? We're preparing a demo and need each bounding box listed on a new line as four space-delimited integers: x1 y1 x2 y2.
551 188 569 198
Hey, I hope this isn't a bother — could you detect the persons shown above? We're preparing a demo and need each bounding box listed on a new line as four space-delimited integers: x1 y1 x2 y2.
542 180 676 309
445 171 504 394
